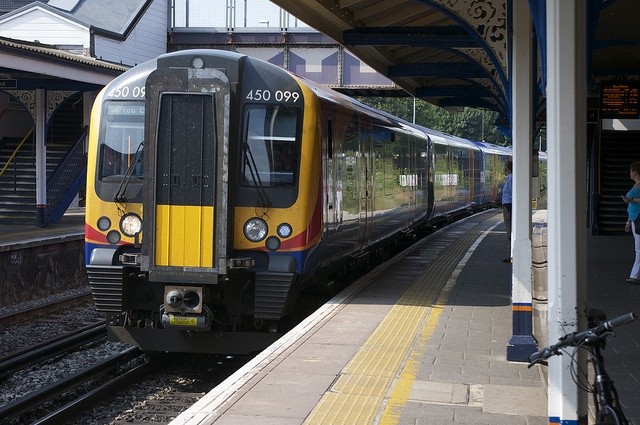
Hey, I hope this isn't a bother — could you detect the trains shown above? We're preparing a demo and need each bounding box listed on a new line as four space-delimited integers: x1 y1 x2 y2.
84 49 547 354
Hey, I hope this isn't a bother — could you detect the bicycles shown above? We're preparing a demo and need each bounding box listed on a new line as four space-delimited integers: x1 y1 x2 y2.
528 309 637 425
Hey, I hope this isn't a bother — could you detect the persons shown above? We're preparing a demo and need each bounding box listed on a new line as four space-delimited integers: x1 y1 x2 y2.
621 163 640 282
501 159 512 263
